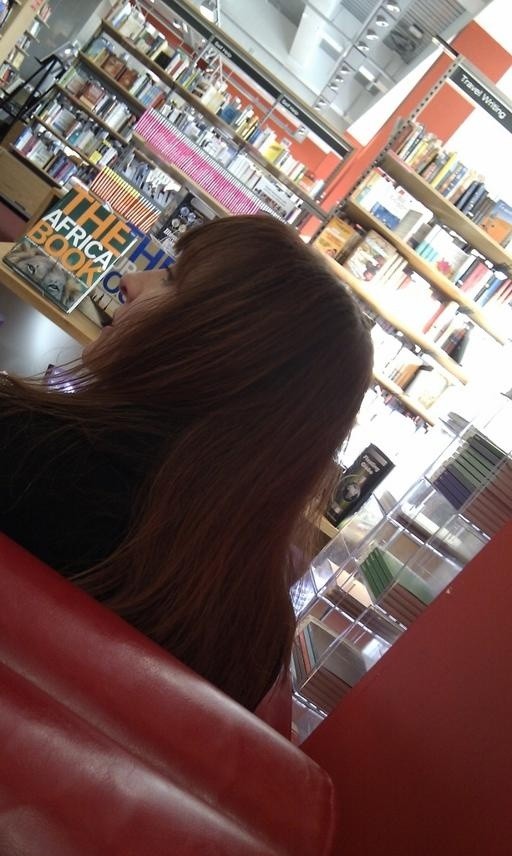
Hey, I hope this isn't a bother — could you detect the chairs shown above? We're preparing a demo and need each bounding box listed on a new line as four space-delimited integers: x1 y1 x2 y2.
1 531 338 856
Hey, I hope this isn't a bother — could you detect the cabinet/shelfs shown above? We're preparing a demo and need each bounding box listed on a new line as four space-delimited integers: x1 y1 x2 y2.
0 0 511 747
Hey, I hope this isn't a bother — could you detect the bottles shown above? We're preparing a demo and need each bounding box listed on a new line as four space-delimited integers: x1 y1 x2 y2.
326 474 366 523
